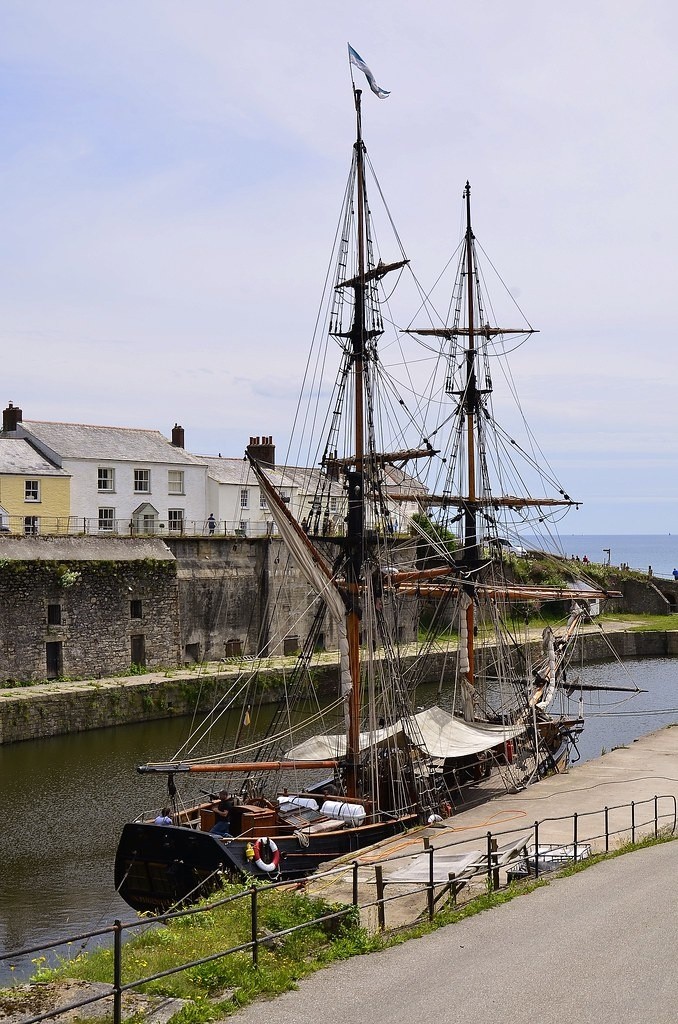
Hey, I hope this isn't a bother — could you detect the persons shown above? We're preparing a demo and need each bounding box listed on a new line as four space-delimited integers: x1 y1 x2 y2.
205 513 217 535
572 555 575 559
575 556 579 561
301 517 307 530
673 568 678 580
209 790 233 838
155 807 173 824
583 555 588 566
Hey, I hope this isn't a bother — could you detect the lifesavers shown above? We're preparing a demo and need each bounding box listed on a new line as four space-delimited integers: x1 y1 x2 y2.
428 814 445 825
251 837 280 872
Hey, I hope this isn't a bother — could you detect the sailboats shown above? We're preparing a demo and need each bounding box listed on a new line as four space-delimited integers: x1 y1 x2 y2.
100 39 655 919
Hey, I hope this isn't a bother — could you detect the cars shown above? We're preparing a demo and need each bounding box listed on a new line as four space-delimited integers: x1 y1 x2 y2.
479 535 527 558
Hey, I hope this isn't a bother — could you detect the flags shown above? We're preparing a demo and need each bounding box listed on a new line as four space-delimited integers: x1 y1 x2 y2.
348 45 391 100
